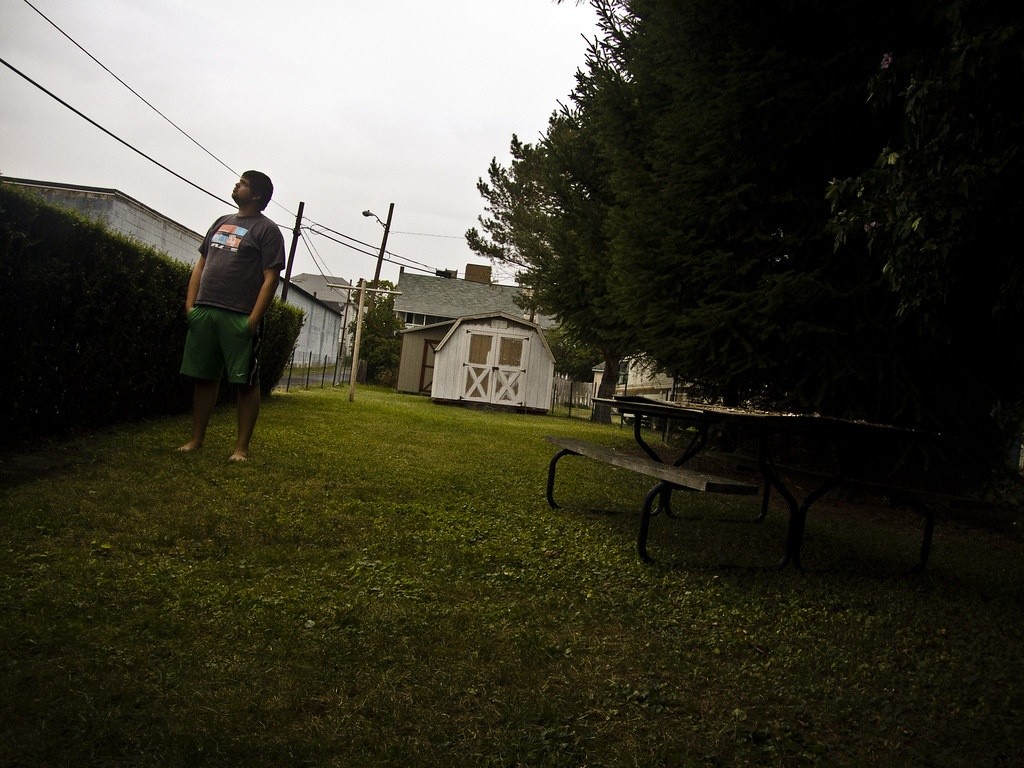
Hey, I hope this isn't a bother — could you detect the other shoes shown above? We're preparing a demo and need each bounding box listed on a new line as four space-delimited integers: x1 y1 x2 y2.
174 440 203 453
227 449 249 462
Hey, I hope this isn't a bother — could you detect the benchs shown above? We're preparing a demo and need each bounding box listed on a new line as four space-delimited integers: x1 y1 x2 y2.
546 436 976 564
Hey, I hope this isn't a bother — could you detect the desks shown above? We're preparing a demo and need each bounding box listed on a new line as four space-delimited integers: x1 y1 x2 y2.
592 397 943 565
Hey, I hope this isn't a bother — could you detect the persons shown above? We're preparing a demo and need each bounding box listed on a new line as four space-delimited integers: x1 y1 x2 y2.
175 171 286 460
